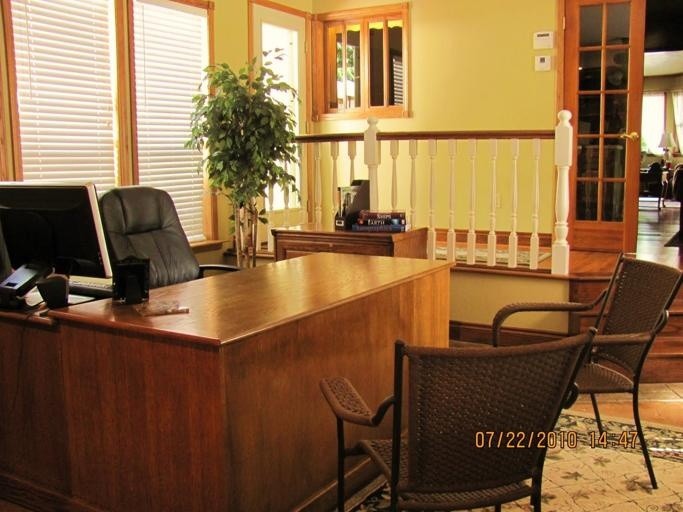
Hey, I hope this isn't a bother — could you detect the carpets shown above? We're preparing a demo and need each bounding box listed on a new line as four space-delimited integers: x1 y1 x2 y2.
345 410 683 511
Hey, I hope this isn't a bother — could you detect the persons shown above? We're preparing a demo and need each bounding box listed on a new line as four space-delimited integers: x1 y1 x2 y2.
655 157 680 211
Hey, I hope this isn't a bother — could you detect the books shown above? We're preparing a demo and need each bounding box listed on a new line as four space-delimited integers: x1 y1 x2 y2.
345 192 357 213
336 186 359 217
357 219 406 225
359 209 405 219
352 224 411 232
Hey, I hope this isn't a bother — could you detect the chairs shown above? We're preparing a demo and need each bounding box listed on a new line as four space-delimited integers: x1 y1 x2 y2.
474 249 683 495
97 184 242 294
320 323 596 512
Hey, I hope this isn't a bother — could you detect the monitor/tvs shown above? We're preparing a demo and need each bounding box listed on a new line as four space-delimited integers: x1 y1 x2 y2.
0 181 119 311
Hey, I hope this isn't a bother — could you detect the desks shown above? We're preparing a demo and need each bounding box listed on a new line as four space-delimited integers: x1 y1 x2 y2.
639 158 669 211
45 248 452 511
0 288 95 508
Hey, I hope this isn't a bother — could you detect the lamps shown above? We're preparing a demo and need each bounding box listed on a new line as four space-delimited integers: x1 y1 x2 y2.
657 131 677 163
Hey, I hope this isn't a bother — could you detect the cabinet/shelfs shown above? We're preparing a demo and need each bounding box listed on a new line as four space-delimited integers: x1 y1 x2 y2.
270 218 429 261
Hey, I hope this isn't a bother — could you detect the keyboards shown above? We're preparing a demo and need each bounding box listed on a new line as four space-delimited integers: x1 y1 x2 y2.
69 276 113 298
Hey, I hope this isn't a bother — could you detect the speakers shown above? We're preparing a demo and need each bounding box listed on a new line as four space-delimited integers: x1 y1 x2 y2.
36 274 68 310
